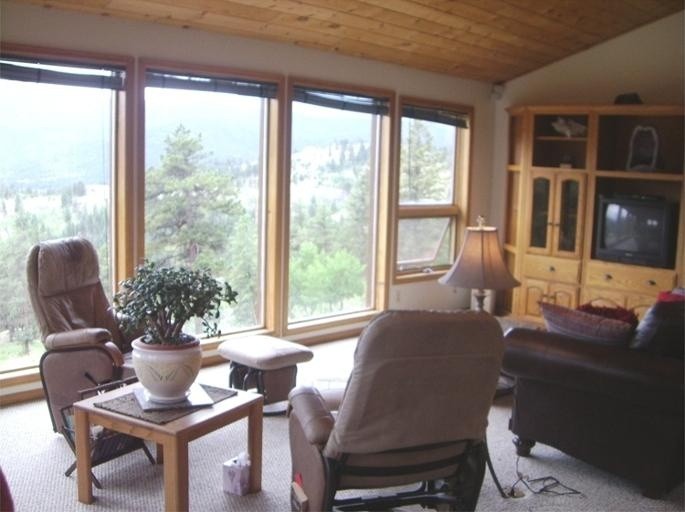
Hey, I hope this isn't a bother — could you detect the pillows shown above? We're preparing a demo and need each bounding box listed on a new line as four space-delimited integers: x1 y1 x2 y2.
535 298 636 343
672 287 685 295
653 291 685 302
577 301 641 332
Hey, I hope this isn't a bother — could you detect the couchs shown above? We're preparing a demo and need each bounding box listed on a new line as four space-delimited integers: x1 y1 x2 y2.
495 286 685 500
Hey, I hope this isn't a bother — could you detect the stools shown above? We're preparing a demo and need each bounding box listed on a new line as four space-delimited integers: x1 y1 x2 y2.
214 333 315 416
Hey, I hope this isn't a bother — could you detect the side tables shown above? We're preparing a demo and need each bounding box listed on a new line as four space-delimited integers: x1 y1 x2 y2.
71 381 265 512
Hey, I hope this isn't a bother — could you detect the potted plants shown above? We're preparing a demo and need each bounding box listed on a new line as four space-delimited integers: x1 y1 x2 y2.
106 259 237 405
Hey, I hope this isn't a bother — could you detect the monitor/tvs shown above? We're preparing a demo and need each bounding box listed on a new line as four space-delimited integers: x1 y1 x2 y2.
593 193 680 270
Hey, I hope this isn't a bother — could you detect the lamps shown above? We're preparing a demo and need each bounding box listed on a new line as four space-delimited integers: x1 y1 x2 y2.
437 213 523 314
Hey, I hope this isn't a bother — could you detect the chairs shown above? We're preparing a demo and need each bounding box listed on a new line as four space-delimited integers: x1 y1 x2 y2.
23 238 156 490
287 308 506 512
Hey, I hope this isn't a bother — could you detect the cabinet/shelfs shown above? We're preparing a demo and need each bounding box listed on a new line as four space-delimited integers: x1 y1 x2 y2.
583 260 679 322
594 105 685 179
504 104 594 320
